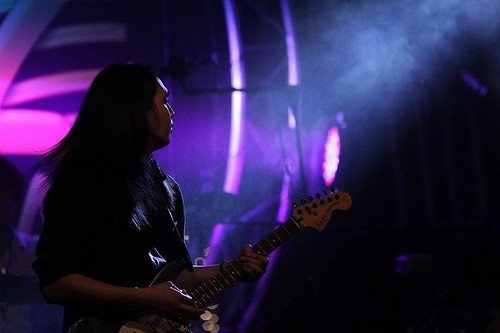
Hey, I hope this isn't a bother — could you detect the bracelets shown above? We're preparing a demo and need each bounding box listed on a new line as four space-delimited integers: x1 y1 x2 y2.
219 260 226 271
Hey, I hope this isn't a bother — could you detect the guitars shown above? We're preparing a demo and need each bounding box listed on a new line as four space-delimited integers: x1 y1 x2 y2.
68 186 353 333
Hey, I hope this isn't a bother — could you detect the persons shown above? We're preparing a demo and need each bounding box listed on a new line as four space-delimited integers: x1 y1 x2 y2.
30 61 271 333
